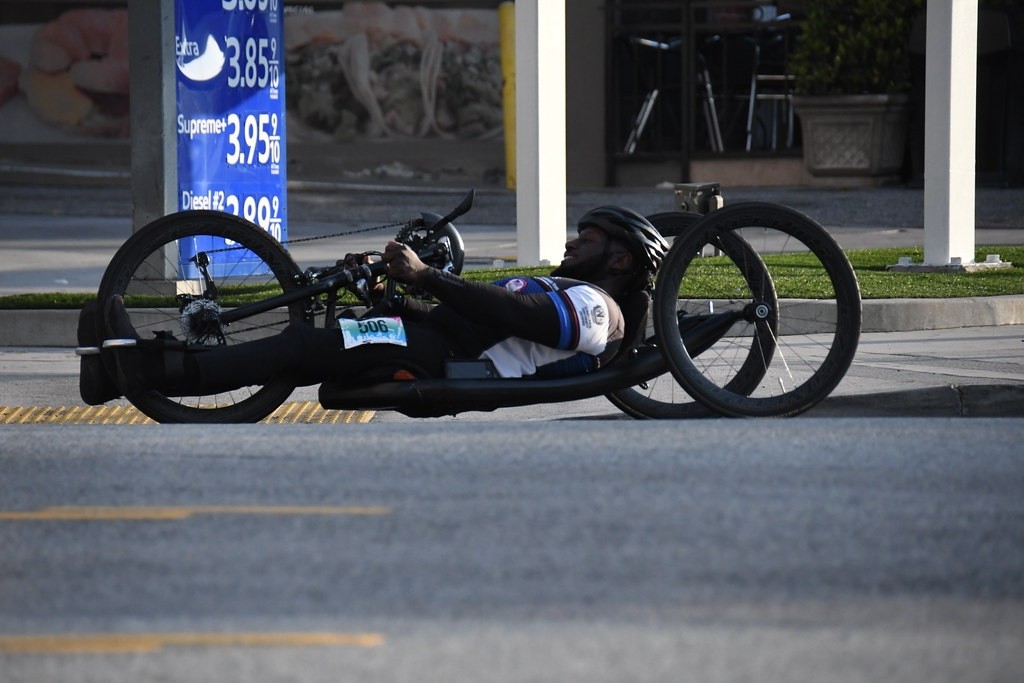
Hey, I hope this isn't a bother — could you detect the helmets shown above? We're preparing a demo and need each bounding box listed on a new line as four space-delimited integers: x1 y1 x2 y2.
578 204 670 275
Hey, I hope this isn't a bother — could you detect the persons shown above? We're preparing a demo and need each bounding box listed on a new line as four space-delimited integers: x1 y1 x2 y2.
76 205 671 406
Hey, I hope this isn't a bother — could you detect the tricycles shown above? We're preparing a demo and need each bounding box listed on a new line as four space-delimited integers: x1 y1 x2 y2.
69 190 867 423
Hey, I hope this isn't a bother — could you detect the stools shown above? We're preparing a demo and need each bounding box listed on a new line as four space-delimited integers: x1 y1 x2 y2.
623 35 723 163
733 31 797 159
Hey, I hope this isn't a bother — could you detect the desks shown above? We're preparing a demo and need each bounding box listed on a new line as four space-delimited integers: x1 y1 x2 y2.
619 18 804 59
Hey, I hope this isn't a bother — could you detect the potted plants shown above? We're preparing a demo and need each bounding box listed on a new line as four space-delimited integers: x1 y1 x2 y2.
784 0 914 182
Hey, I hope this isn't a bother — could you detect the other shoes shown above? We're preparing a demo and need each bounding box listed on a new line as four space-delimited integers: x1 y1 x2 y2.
105 295 158 391
76 296 124 407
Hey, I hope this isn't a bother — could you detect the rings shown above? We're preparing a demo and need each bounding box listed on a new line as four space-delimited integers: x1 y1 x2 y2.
386 263 392 270
